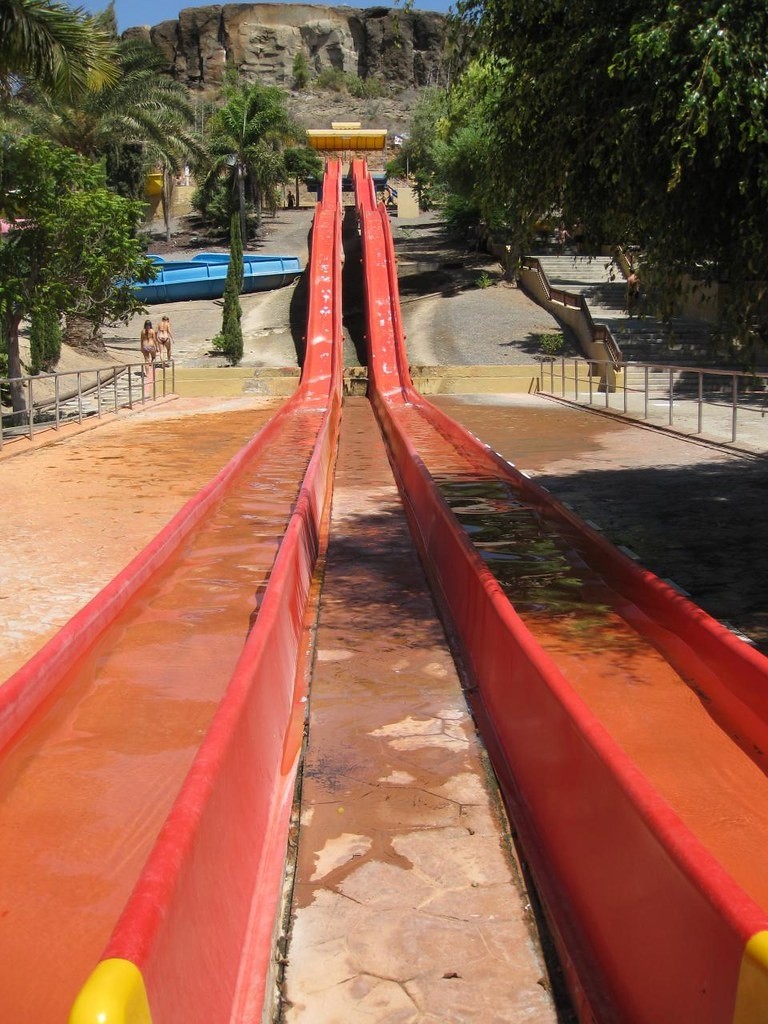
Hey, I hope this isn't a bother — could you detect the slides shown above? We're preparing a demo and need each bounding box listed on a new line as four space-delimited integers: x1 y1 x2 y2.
1 157 767 1024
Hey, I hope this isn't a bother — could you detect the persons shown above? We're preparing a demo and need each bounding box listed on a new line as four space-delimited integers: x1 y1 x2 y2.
156 316 174 367
141 320 159 376
555 217 642 320
286 190 296 208
381 187 396 205
